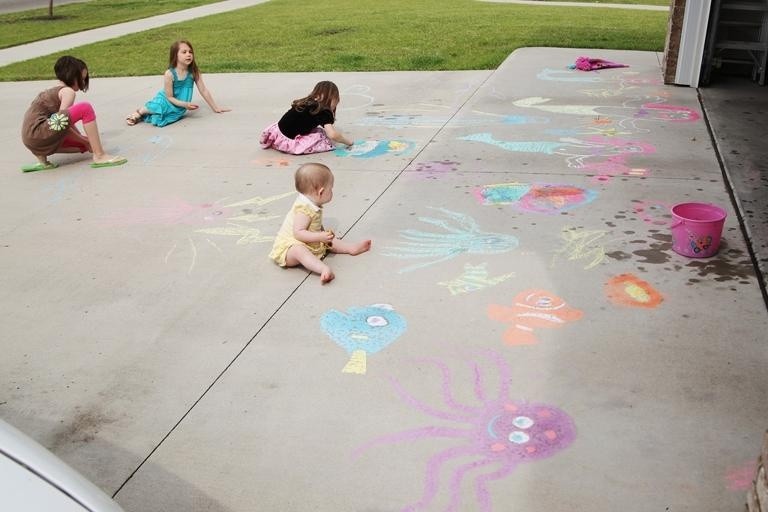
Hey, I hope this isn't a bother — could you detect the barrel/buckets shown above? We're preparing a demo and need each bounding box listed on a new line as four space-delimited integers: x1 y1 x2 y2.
669 201 727 258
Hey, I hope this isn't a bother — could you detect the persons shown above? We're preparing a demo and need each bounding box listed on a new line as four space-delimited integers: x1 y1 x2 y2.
19 54 127 169
125 38 232 127
258 80 354 156
268 161 371 283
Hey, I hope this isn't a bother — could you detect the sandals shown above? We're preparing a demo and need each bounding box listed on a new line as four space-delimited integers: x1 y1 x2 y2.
127 110 145 125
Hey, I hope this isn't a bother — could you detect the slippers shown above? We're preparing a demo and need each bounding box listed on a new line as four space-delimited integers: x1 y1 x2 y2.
91 157 127 167
22 161 57 172
